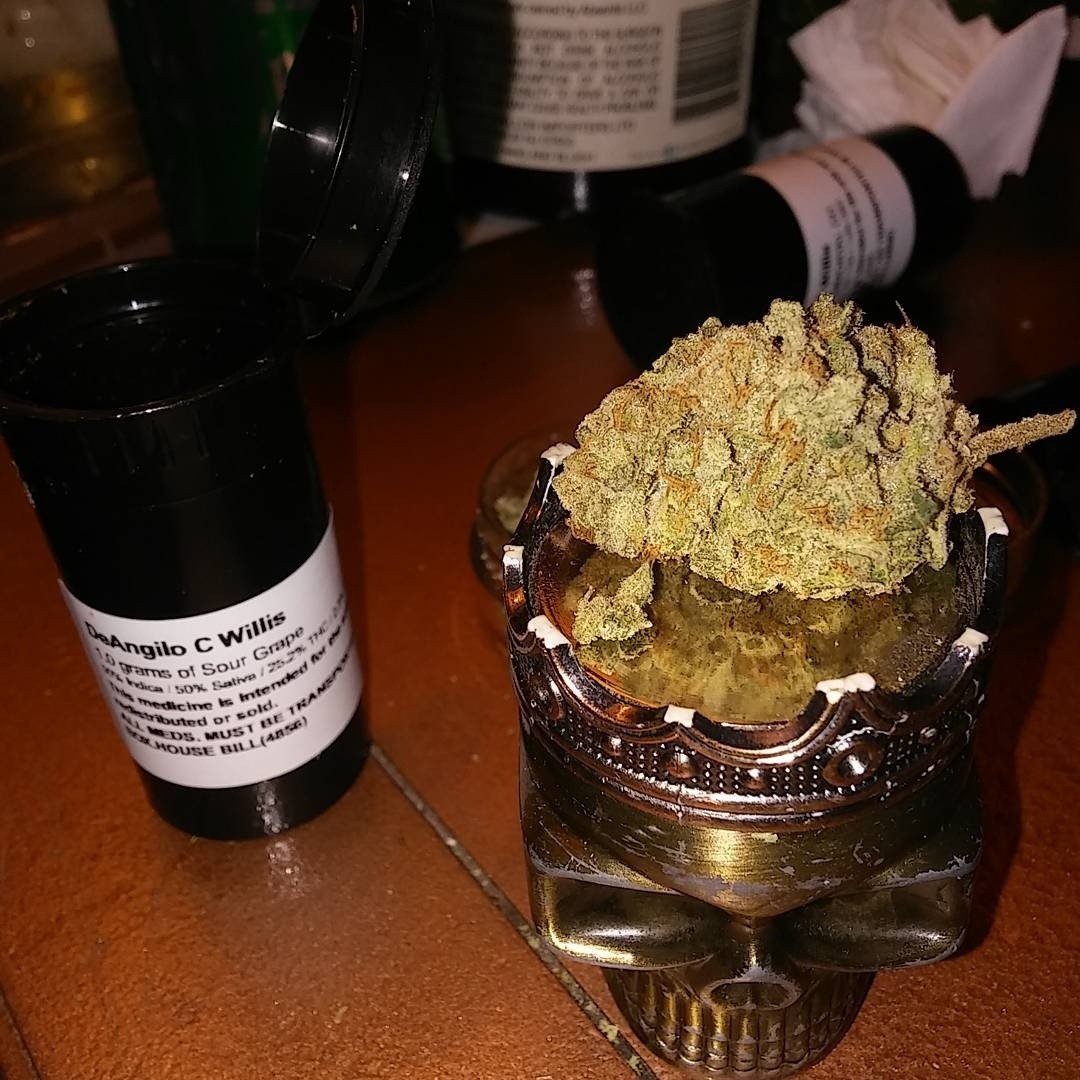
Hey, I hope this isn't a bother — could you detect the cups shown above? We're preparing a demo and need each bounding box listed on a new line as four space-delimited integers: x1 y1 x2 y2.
595 127 972 373
4 255 365 844
451 0 758 223
